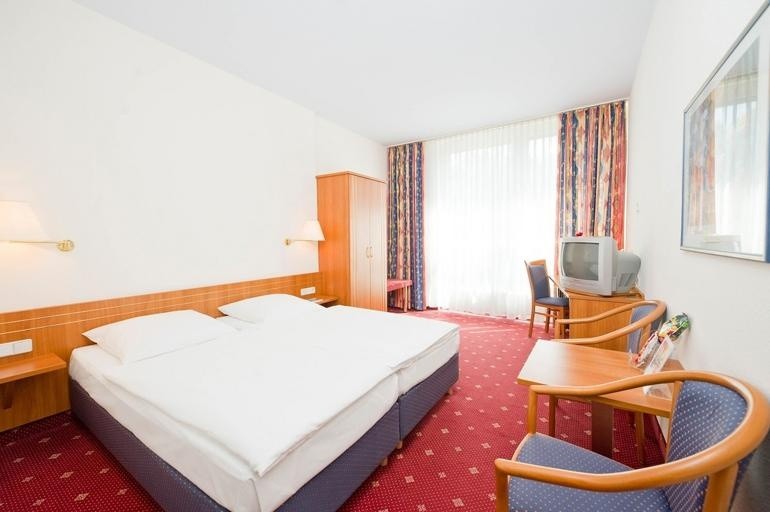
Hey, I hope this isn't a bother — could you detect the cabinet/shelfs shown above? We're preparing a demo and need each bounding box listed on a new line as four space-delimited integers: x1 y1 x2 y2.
0 352 66 431
315 171 388 313
308 296 337 308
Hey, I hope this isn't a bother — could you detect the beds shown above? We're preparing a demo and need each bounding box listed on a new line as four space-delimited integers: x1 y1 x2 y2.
70 319 400 511
214 307 462 445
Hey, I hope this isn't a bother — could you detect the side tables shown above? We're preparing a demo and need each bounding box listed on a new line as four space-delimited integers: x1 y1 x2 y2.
519 339 687 437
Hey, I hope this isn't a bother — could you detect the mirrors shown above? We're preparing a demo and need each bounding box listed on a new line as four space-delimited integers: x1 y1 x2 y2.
680 0 770 263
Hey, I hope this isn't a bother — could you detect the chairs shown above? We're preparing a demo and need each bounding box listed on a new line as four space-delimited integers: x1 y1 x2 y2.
525 259 568 338
494 370 770 512
555 300 668 352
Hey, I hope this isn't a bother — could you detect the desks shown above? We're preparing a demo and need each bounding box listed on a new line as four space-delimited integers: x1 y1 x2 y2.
549 274 643 350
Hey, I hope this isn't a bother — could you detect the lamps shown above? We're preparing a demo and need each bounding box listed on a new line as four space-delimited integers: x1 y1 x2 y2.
286 220 324 245
1 201 73 250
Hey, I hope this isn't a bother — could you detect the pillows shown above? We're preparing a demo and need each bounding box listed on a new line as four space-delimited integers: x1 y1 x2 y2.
220 293 324 324
81 310 237 360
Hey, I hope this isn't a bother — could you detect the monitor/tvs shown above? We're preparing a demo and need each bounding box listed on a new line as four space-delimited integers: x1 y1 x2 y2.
557 236 641 297
